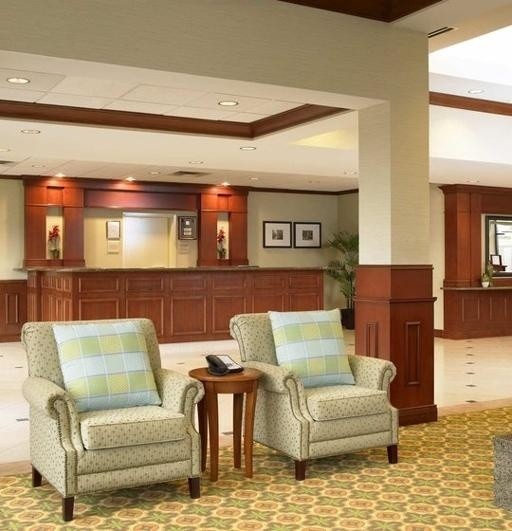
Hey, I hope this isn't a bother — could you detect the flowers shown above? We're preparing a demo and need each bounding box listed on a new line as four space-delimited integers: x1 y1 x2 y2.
216 229 227 255
47 225 62 251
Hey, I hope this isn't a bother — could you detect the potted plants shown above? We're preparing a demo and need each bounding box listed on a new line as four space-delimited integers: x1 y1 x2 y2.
479 273 490 289
321 230 359 331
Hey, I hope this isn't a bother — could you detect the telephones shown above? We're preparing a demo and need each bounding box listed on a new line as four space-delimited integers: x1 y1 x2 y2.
206 355 245 373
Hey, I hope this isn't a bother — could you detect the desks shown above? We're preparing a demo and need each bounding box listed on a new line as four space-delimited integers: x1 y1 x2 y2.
188 368 266 484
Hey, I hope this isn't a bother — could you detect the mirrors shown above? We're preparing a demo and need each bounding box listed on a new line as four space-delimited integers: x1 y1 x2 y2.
483 215 512 278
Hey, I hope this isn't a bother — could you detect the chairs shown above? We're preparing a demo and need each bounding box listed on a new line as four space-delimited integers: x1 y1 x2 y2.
228 309 402 486
21 318 209 529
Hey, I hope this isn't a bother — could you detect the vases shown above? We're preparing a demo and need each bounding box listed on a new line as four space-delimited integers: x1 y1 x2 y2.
52 250 60 261
219 252 225 260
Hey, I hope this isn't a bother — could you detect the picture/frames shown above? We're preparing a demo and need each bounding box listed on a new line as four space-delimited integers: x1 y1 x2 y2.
177 216 198 241
105 221 121 241
262 220 322 250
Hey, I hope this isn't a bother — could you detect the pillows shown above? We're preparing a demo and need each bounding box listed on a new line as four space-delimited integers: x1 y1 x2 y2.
267 308 359 389
50 320 164 415
